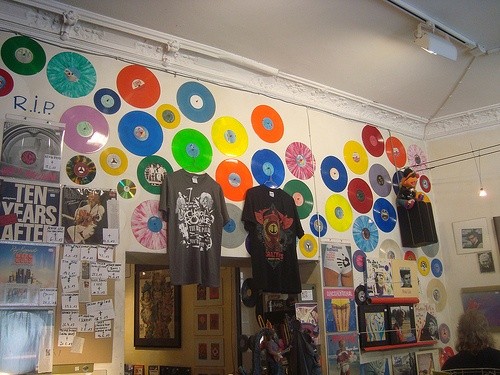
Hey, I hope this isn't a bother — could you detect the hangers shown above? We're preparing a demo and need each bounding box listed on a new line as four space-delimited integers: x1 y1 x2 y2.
182 151 206 173
264 167 279 188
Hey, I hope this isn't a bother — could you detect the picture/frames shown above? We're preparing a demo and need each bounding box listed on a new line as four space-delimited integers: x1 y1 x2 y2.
194 369 225 375
359 303 417 347
194 338 224 366
193 308 224 335
133 264 181 348
193 278 223 306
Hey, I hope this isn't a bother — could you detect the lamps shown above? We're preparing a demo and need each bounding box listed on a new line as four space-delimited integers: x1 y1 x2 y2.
474 157 488 197
415 22 458 61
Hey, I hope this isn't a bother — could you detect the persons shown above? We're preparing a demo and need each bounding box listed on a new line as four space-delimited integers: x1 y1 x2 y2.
467 232 483 248
479 254 493 271
336 339 352 375
68 192 104 244
441 310 500 375
400 270 412 288
